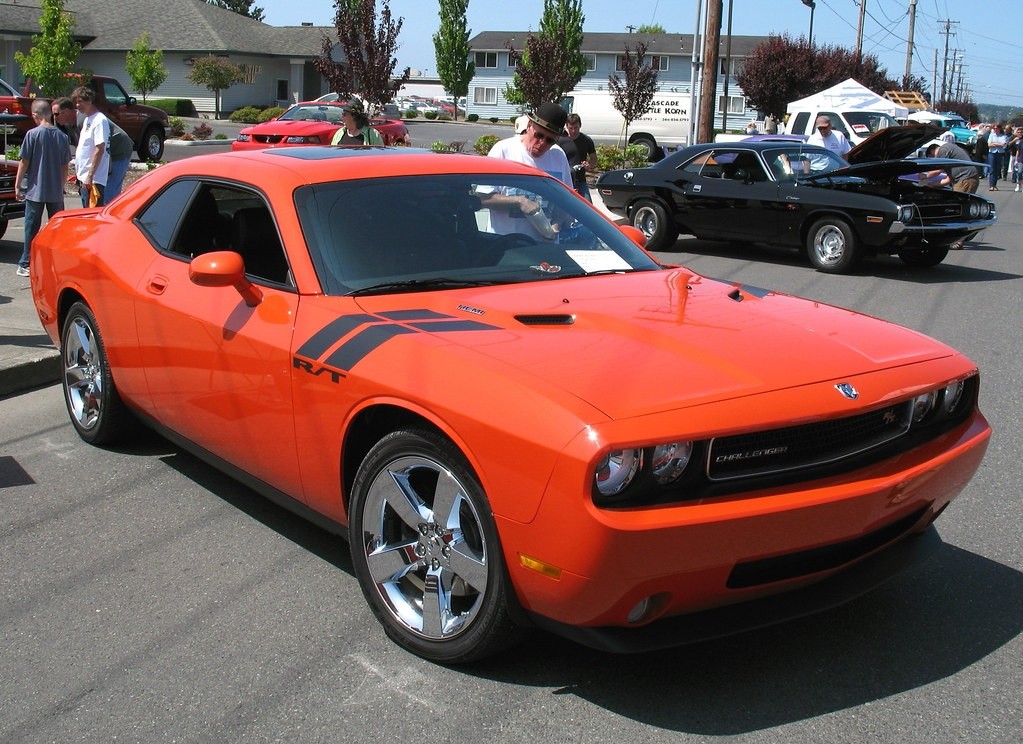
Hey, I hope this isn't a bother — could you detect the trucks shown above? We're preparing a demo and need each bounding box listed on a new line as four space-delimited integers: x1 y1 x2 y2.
908 109 979 145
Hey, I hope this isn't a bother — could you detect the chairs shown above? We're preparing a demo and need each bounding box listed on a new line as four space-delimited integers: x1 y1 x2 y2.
171 188 290 290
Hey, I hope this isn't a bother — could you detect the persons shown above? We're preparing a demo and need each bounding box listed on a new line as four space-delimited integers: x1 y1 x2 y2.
51 97 135 205
515 114 597 204
975 123 1023 192
330 99 383 146
763 113 778 135
70 86 110 208
803 116 851 174
475 102 574 244
14 99 73 276
918 143 979 249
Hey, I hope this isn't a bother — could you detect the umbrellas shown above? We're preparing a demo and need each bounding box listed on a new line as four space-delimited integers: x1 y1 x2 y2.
906 110 965 126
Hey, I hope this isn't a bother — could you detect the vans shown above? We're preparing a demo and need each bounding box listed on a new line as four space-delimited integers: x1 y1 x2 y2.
515 89 692 162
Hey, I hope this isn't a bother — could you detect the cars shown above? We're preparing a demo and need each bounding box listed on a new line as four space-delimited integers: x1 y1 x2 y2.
29 142 992 666
661 123 952 187
388 95 467 115
595 141 998 273
233 100 413 151
288 91 402 121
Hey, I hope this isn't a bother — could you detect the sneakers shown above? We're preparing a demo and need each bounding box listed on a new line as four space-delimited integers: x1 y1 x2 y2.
16 266 31 277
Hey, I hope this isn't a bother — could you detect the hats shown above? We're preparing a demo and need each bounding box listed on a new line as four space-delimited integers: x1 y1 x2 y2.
515 115 529 134
816 116 831 128
340 98 369 121
527 102 568 138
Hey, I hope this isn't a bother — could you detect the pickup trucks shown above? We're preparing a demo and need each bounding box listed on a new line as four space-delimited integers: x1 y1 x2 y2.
0 72 169 164
715 107 948 161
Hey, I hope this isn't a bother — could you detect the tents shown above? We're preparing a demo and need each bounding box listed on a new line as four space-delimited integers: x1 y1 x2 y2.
787 78 909 129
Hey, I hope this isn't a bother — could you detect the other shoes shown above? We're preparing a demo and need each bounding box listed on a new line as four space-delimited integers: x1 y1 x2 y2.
988 186 994 191
993 185 997 191
1015 185 1022 191
950 243 964 250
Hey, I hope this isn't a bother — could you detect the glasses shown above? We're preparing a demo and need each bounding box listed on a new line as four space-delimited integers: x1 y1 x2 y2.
53 110 67 117
531 123 556 145
341 111 348 117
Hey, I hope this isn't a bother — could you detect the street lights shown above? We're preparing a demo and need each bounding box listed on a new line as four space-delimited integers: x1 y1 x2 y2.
803 0 815 46
